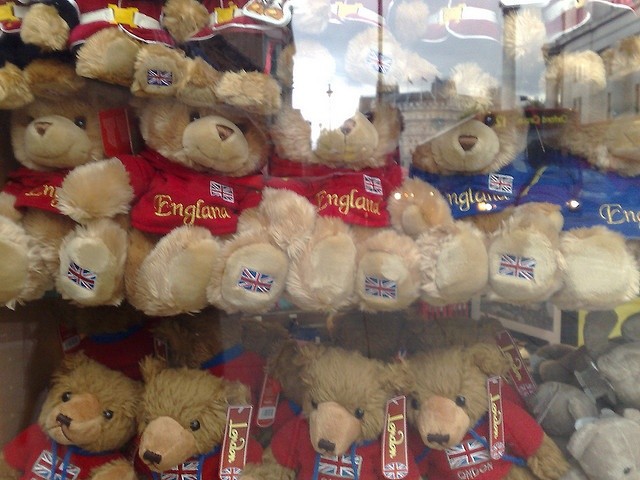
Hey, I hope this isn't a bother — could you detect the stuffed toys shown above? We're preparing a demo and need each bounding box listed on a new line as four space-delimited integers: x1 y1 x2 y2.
0 66 140 312
408 316 504 352
1 339 147 480
254 317 425 480
327 303 408 361
65 0 206 100
389 88 555 313
385 2 544 118
1 0 87 111
126 68 286 314
521 0 640 110
382 338 566 480
525 307 640 479
242 335 319 447
522 86 640 305
157 315 267 390
278 2 408 102
257 81 440 318
157 0 286 116
130 316 266 480
520 93 568 167
58 305 152 384
